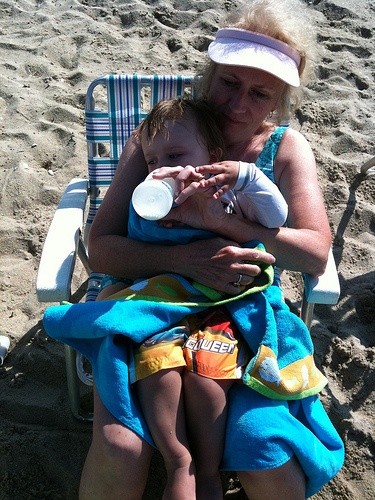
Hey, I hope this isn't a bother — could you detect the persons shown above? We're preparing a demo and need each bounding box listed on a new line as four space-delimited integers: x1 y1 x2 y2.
78 0 332 500
128 97 289 500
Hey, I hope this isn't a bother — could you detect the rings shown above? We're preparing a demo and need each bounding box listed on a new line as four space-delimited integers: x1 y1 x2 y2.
233 274 244 287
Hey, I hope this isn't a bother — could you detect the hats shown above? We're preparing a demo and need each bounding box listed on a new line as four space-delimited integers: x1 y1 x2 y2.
208 28 301 87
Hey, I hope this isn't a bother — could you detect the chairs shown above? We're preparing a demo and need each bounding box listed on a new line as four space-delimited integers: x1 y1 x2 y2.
36 75 340 425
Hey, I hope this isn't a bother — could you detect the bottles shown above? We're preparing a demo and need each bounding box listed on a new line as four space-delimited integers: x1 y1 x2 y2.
131 167 177 221
